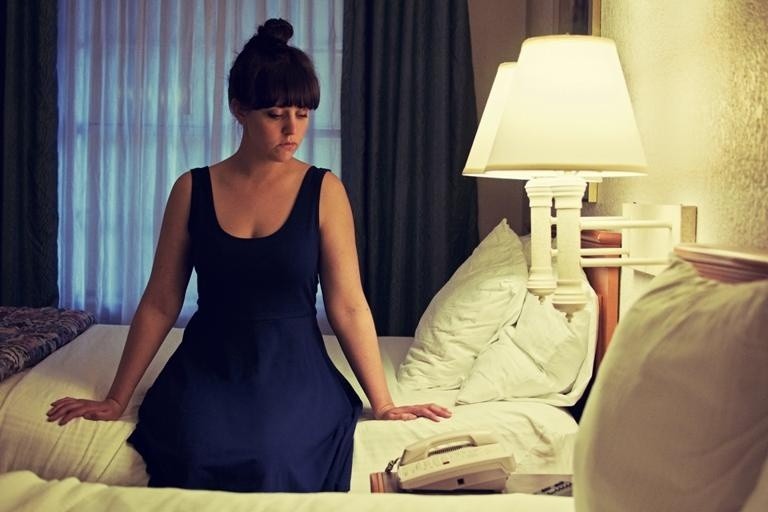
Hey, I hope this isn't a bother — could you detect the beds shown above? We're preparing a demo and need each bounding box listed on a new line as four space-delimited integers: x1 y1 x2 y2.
0 219 623 491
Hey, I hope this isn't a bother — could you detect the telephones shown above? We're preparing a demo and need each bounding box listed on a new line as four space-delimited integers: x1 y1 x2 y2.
396 428 517 493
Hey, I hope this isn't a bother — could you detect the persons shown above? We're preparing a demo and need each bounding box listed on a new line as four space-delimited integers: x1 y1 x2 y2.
47 17 453 493
